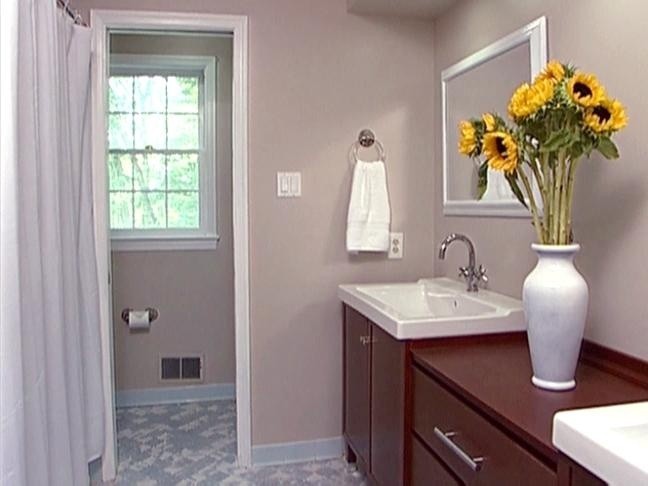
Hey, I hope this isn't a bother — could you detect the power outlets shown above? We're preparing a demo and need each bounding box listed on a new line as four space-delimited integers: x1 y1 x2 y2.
387 231 404 260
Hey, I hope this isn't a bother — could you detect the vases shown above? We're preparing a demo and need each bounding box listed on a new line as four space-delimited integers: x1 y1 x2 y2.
522 243 588 390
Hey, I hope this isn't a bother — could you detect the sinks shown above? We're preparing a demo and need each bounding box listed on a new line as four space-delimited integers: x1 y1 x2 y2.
337 277 527 342
551 399 647 486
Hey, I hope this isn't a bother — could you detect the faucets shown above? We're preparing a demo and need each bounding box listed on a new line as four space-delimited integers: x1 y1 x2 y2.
438 233 489 292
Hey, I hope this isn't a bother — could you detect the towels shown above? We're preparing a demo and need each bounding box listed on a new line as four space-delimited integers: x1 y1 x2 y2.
477 160 516 203
345 160 394 254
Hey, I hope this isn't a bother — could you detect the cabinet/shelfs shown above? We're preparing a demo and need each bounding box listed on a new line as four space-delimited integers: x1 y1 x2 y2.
342 302 527 486
409 361 560 486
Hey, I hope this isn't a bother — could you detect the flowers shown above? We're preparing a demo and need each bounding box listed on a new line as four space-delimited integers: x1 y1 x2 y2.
460 59 628 245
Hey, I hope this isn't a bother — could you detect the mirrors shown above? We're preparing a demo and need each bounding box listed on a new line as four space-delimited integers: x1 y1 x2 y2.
443 15 548 219
